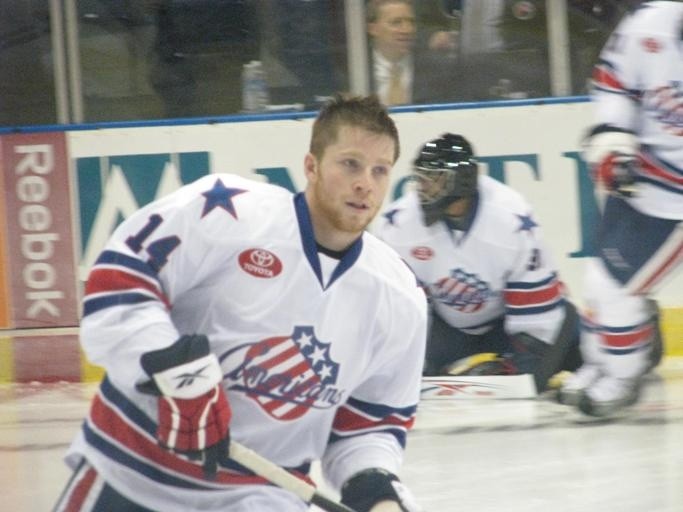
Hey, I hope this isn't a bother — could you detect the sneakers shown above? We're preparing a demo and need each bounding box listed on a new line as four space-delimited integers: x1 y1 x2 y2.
556 361 641 404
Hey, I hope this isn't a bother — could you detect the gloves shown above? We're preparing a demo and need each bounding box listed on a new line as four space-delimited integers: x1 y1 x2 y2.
604 153 645 199
142 335 232 477
342 468 415 512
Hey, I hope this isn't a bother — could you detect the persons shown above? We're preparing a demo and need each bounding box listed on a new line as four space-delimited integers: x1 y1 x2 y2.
576 1 683 417
373 132 582 392
366 1 631 105
51 91 429 511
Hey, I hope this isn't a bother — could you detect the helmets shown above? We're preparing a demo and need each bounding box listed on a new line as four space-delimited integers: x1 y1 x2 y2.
416 133 478 227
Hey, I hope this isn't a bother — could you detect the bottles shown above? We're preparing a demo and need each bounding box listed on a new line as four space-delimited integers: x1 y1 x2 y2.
240 60 267 112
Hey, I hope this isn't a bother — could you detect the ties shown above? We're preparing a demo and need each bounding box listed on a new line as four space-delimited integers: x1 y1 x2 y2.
387 65 407 107
